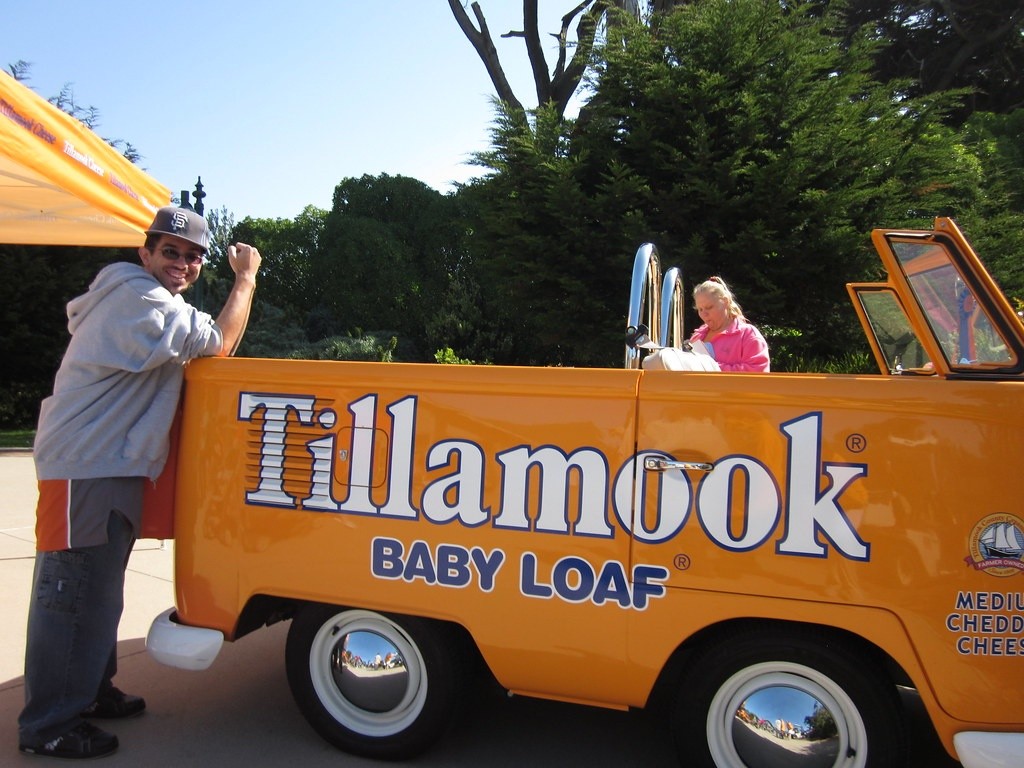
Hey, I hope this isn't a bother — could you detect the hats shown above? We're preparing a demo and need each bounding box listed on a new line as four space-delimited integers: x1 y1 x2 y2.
143 206 212 252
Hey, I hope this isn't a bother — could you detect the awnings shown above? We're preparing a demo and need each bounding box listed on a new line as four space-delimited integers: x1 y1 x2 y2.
0 68 170 248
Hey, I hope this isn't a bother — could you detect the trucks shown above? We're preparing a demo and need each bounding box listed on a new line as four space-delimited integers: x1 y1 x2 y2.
136 208 1024 768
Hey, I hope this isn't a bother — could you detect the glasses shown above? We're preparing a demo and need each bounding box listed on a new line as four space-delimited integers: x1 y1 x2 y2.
147 244 205 266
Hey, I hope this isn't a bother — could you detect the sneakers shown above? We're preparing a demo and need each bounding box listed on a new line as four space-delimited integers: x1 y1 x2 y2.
81 683 147 721
17 724 120 761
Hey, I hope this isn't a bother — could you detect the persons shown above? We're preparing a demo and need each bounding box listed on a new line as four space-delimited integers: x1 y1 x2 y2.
19 206 261 758
687 277 769 374
